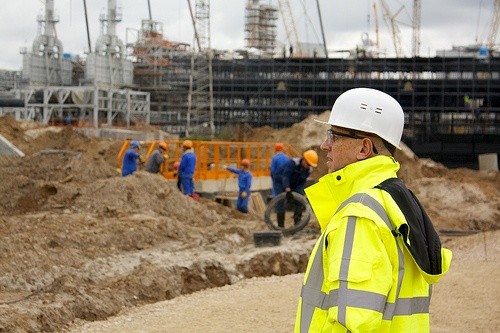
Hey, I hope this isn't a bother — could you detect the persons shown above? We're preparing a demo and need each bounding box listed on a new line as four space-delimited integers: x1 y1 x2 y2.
294 88 453 333
223 159 252 214
147 143 168 174
269 144 292 195
274 150 318 229
122 141 145 177
178 140 196 195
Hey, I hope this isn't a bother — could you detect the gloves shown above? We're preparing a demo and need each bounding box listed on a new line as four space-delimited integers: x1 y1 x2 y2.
286 191 292 202
222 166 227 169
241 192 247 198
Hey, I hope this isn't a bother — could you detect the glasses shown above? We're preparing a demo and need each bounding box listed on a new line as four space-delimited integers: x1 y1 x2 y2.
325 129 378 154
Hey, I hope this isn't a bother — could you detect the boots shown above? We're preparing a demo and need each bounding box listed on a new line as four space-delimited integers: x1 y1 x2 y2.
294 206 303 225
276 212 284 228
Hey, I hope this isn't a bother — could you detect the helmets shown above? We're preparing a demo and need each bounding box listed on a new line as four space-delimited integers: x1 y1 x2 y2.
182 140 192 147
130 141 139 149
159 143 166 150
274 144 283 151
312 88 405 151
240 159 250 165
303 150 318 167
